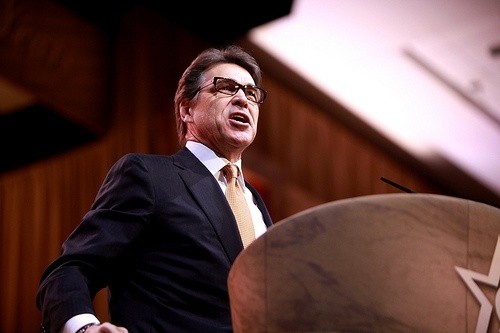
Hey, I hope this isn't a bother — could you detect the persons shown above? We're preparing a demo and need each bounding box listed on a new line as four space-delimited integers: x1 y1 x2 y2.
35 46 272 333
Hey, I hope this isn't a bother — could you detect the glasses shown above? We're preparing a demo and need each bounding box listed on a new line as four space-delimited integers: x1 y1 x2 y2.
187 76 266 104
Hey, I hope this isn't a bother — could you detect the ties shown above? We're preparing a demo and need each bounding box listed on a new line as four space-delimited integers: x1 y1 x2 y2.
221 164 256 249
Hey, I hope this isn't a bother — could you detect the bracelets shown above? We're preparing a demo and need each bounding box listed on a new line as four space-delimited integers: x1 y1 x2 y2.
77 324 94 333
